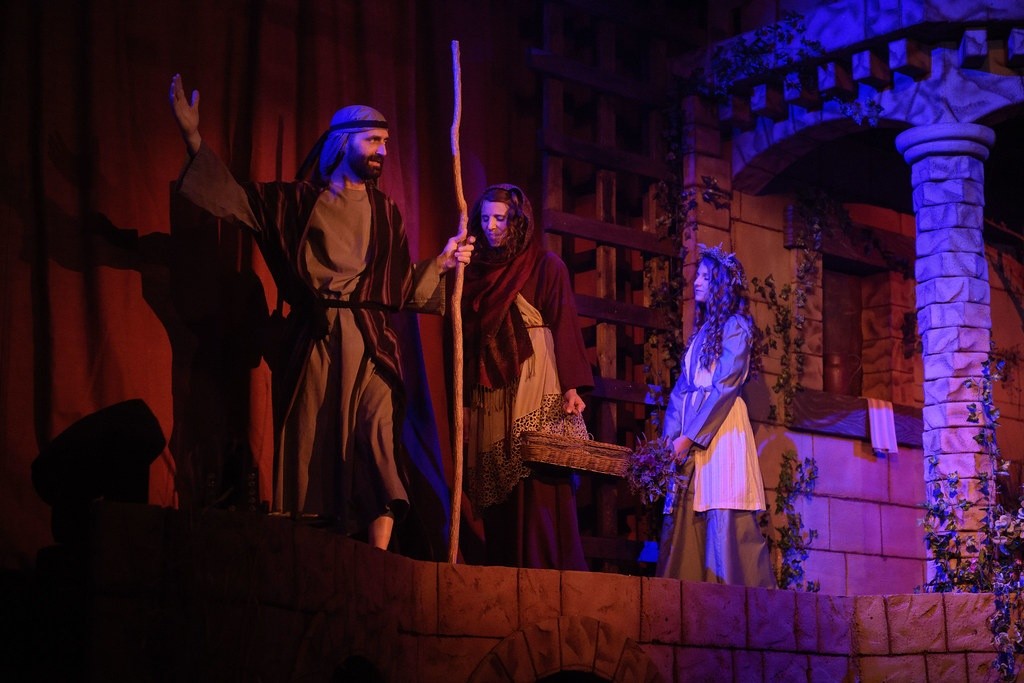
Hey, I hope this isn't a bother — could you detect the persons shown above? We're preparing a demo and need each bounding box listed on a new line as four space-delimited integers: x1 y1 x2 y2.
450 185 589 572
168 71 476 551
653 243 781 591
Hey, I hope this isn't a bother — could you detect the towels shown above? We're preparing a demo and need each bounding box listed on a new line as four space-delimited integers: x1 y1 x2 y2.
854 396 900 456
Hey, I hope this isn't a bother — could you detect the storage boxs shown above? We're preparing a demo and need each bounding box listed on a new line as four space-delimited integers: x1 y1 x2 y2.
521 431 632 478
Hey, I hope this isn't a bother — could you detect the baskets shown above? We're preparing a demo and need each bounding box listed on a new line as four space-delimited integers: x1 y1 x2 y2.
520 430 631 478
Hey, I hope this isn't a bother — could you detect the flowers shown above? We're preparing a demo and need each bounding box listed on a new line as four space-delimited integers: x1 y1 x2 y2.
627 440 684 504
